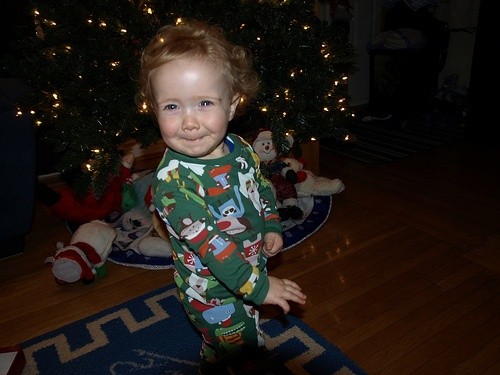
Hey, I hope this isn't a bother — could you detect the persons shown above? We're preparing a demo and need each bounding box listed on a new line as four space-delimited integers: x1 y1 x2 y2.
134 23 309 374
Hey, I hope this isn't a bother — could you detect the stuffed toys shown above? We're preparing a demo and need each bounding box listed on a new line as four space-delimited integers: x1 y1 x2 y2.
49 218 117 285
37 153 133 227
248 129 307 219
281 159 345 199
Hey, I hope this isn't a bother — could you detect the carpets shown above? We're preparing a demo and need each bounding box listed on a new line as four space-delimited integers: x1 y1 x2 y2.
16 282 368 375
66 170 332 270
318 104 472 166
